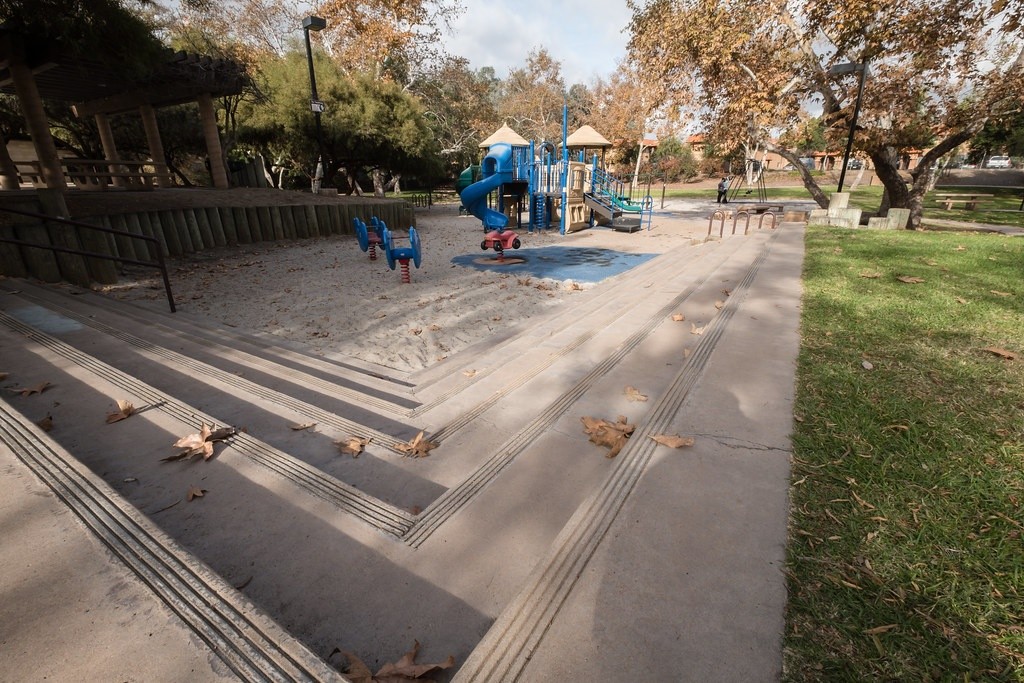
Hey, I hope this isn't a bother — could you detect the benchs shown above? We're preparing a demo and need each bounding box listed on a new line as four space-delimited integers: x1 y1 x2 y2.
754 203 785 212
735 205 771 214
928 199 995 210
783 210 809 222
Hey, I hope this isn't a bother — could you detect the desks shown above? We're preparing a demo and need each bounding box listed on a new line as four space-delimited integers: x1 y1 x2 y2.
933 193 993 207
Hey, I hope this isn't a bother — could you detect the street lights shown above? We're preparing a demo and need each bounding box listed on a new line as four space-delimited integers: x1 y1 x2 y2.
300 15 336 189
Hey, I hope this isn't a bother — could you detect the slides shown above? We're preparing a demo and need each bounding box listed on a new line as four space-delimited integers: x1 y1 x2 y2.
456 163 483 211
602 189 641 211
461 173 509 229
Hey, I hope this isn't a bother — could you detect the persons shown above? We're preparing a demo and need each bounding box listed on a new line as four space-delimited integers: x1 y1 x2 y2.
722 175 733 203
717 177 726 202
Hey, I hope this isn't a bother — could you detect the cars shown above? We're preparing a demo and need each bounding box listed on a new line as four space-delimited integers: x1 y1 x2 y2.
794 157 815 171
986 155 1012 168
847 159 863 170
